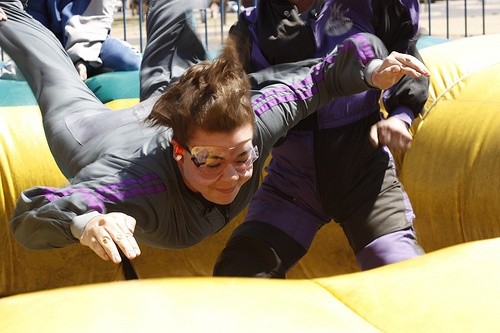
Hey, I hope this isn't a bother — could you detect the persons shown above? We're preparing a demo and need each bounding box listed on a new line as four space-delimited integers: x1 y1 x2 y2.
1 0 143 84
1 1 430 263
211 0 429 280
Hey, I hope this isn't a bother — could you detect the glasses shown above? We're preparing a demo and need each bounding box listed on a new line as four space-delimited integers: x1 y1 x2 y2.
182 145 259 177
